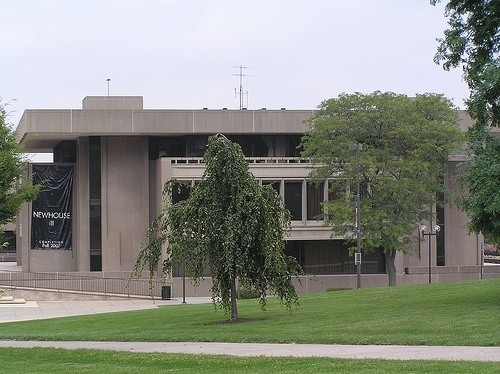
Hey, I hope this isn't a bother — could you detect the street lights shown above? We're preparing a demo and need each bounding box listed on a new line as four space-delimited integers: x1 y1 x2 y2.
420 224 441 283
349 141 366 289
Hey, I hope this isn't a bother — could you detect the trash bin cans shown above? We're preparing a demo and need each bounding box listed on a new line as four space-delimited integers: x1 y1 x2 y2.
160 285 171 300
